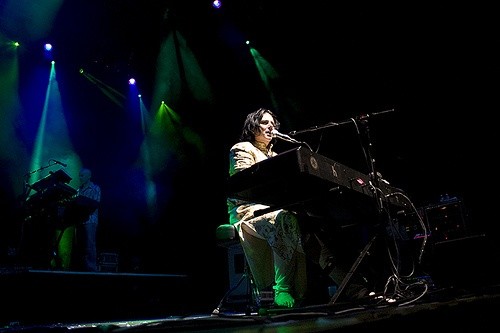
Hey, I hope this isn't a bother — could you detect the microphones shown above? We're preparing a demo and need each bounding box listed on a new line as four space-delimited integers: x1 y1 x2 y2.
270 129 296 144
53 159 67 167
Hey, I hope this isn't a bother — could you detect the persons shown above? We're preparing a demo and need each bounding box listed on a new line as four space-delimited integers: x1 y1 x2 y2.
72 168 101 272
227 107 356 308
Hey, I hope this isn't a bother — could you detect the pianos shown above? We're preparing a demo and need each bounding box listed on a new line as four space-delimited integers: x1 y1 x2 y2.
24 181 77 217
41 195 98 230
218 147 404 212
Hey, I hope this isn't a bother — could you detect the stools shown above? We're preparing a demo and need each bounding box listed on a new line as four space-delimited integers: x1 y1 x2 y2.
211 223 266 316
